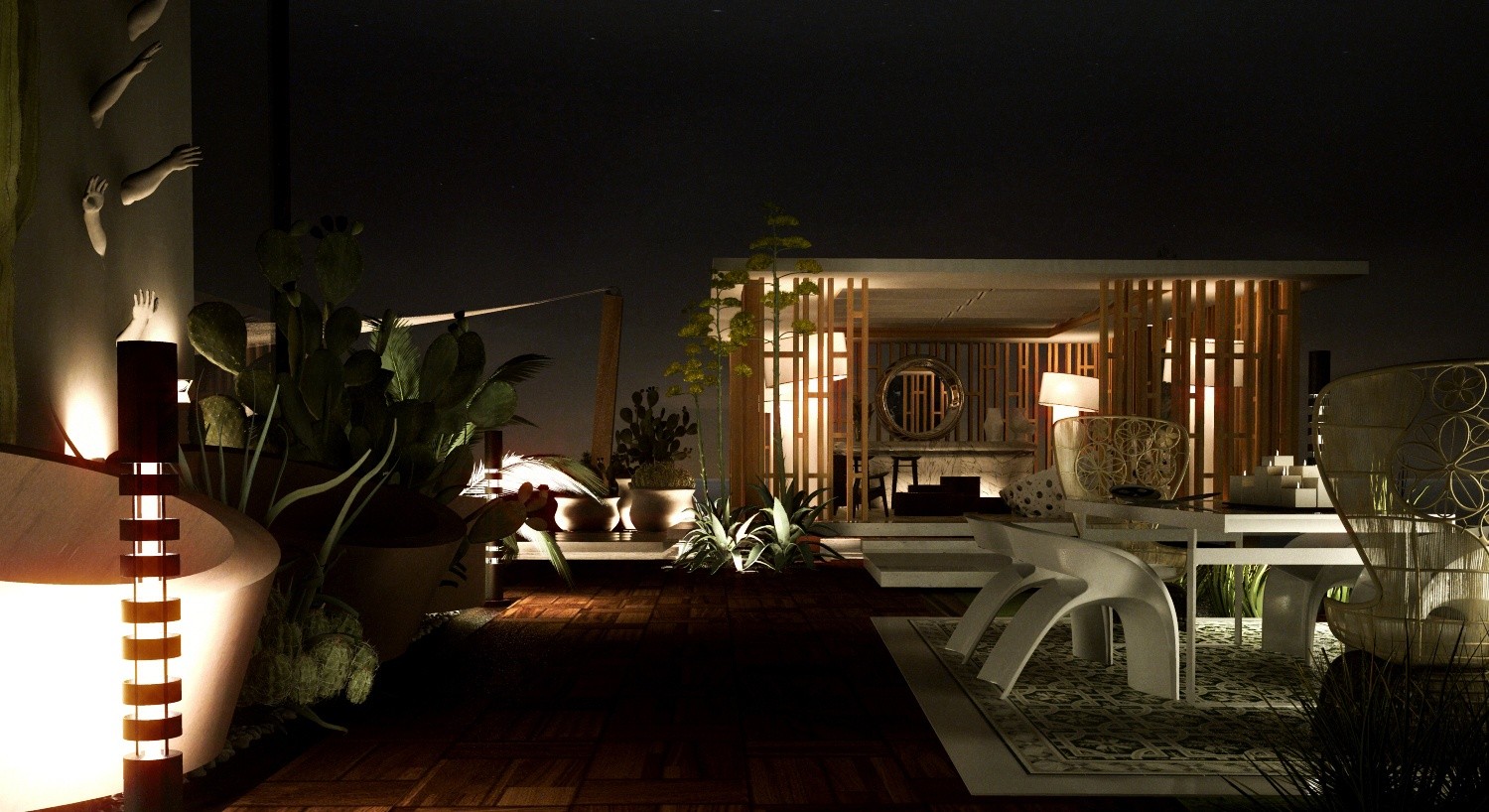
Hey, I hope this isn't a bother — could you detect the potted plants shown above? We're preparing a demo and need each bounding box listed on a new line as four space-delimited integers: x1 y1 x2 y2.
552 483 622 531
626 464 697 536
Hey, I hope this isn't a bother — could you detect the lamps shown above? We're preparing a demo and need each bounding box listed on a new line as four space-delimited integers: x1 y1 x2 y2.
118 338 194 812
1038 372 1102 497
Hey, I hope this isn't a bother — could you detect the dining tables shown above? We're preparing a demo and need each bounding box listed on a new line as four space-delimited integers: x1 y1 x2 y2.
1062 494 1459 689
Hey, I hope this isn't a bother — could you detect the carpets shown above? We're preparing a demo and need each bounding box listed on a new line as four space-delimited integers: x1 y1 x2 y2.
869 611 1458 796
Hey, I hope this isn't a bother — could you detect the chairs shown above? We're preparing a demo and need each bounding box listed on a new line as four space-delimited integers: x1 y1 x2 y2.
944 511 1103 666
978 521 1181 706
1264 534 1386 666
852 454 889 520
1349 526 1489 672
